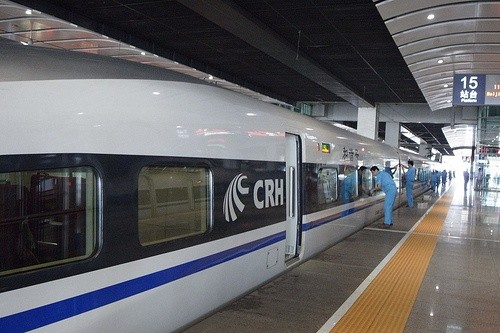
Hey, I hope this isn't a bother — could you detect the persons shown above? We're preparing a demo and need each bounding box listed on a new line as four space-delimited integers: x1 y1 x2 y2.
384 161 399 179
474 168 482 192
400 160 416 208
420 169 433 189
463 169 469 190
340 166 367 219
485 174 491 188
429 170 453 191
369 166 398 228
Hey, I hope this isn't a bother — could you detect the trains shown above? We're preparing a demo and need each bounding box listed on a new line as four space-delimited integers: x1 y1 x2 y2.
1 37 442 332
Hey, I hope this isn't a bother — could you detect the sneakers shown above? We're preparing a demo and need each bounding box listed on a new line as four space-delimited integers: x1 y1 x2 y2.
378 223 390 228
